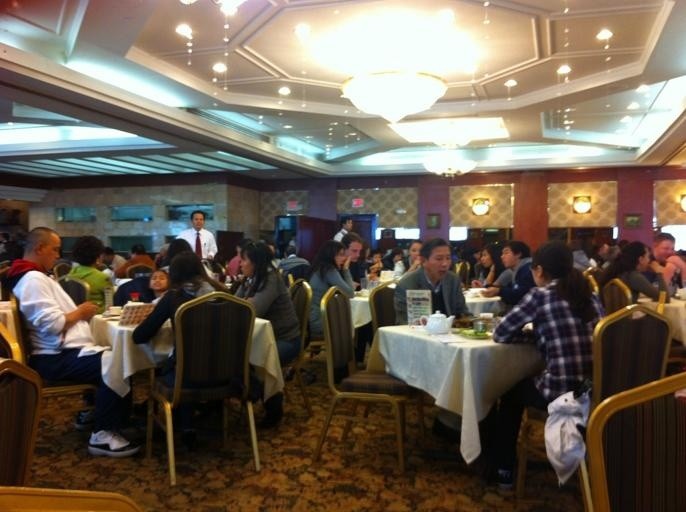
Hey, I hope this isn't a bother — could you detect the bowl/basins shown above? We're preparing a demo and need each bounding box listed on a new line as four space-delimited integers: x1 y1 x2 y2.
470 287 485 296
479 312 493 319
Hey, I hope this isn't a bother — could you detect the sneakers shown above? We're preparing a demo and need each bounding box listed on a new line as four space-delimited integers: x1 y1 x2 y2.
496 468 514 490
74 406 98 431
88 430 141 457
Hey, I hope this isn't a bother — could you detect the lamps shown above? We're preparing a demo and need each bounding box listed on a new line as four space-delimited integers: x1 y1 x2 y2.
472 198 492 218
573 193 594 214
340 70 449 124
422 155 478 179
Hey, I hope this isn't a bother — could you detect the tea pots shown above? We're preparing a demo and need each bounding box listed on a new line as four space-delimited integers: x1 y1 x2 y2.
379 269 396 283
419 310 456 334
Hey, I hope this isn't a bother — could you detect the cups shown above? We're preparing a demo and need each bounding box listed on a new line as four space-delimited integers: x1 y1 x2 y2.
109 306 121 316
368 281 377 293
360 278 369 291
474 320 488 336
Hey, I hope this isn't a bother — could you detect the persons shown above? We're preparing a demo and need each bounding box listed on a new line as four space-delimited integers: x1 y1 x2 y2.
233 242 301 429
572 234 686 304
492 240 607 489
304 240 355 340
175 210 218 267
333 217 354 243
168 239 192 261
132 251 233 453
360 239 424 281
214 239 311 278
65 235 114 314
394 239 475 439
450 240 538 308
6 227 141 458
342 232 374 370
104 245 169 298
0 233 16 255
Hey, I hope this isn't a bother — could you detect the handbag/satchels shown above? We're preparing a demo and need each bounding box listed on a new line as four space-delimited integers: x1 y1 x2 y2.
294 366 318 386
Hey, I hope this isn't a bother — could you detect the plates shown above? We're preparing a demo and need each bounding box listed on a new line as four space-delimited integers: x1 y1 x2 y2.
461 331 493 339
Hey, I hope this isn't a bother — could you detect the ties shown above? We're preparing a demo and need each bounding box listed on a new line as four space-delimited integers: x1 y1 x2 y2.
196 233 201 261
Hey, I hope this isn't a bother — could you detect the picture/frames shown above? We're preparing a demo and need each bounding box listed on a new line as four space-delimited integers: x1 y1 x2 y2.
622 213 642 229
426 213 441 229
